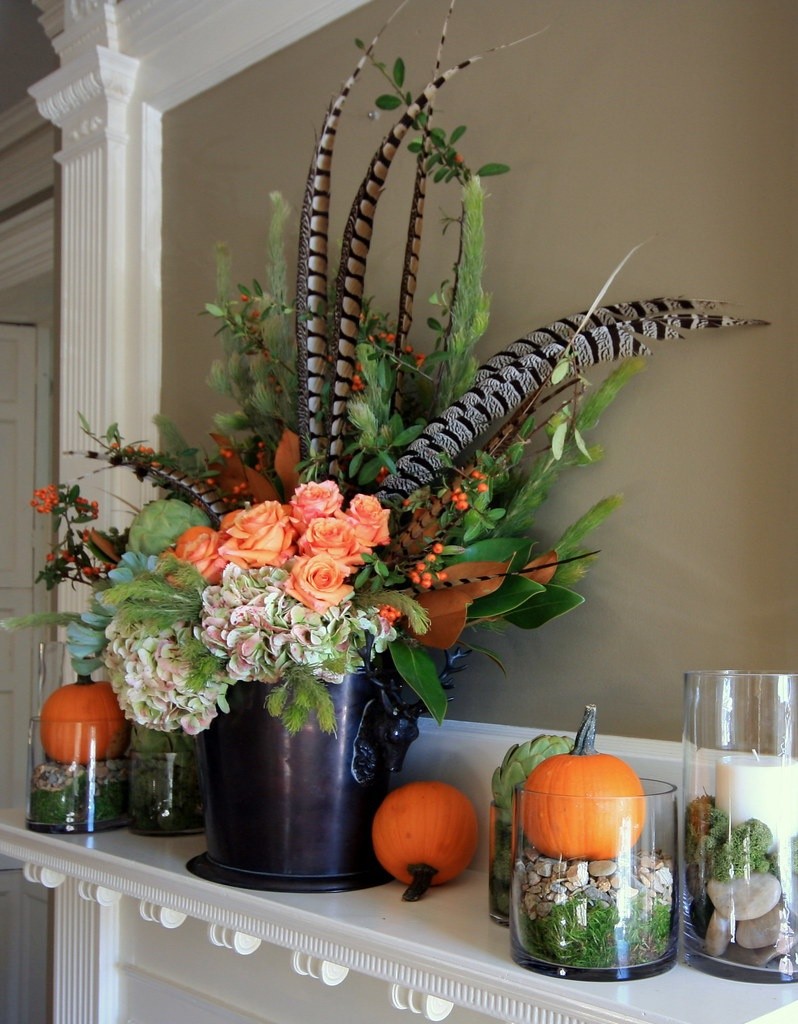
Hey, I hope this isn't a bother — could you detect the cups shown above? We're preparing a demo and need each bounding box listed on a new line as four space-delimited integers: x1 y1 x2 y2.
25 713 133 834
131 750 206 835
679 669 798 983
508 777 679 980
489 801 510 927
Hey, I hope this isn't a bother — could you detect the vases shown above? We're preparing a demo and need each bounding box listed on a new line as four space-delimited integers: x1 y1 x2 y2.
185 644 471 895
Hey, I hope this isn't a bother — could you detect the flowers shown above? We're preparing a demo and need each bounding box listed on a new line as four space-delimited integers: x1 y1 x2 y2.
0 0 774 749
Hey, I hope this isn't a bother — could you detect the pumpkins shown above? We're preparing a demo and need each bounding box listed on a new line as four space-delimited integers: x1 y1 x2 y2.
372 782 478 900
40 656 129 764
524 705 645 857
